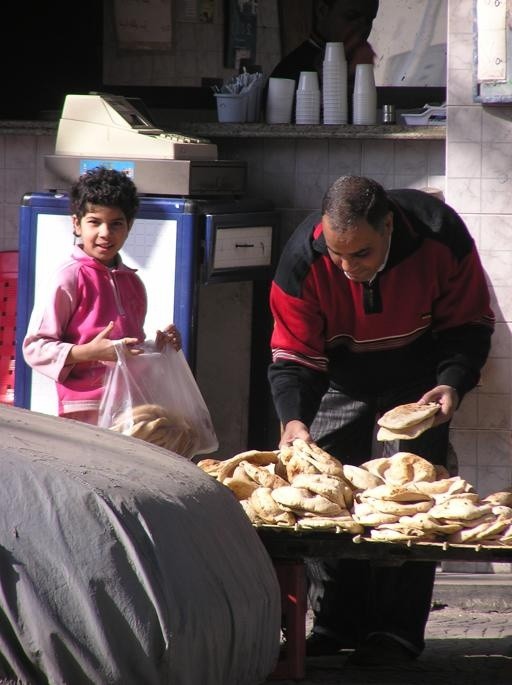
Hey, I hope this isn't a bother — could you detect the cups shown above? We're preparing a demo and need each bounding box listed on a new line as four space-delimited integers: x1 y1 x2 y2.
295 71 319 126
264 76 296 125
321 40 349 127
353 62 378 127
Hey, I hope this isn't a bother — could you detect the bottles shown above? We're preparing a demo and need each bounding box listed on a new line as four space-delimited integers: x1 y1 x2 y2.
381 104 396 125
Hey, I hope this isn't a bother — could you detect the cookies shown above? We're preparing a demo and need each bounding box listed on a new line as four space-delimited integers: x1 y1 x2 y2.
108 404 197 458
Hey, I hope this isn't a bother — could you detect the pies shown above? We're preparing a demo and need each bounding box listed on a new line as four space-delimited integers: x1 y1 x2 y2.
196 438 512 545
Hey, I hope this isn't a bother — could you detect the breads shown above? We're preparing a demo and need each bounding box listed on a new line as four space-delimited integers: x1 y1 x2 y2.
377 401 441 442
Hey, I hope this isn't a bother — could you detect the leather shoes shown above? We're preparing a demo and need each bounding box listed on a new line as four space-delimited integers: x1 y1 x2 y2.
347 637 411 664
279 631 336 655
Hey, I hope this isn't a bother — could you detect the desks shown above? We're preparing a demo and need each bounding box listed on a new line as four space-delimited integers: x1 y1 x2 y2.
244 528 510 684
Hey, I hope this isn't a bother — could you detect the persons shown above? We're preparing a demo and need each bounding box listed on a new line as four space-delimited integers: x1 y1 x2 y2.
262 0 380 96
19 165 182 430
260 172 496 666
